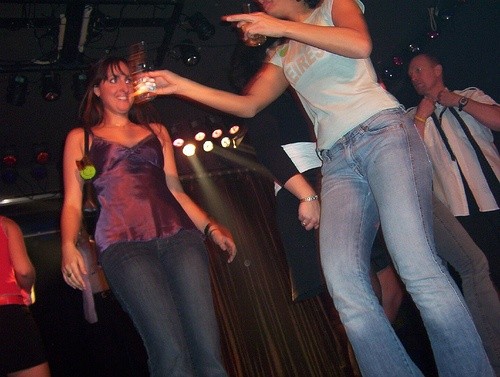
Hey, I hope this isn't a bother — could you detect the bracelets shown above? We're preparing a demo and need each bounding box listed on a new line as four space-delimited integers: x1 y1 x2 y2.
300 194 318 202
204 221 220 237
414 115 426 123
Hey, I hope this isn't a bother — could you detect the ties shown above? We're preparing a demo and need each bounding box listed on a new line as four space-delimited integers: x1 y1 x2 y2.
430 106 500 218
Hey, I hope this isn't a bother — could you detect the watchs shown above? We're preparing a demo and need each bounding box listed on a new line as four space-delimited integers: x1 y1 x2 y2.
458 96 469 111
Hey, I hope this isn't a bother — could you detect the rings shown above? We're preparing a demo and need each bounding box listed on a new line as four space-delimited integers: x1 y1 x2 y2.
68 272 74 277
302 221 306 226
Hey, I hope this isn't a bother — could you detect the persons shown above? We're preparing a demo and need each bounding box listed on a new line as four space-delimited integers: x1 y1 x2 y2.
0 216 52 377
136 0 500 377
60 54 238 377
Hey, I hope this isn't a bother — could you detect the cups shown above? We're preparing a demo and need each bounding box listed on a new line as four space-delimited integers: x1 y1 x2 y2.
128 65 153 104
233 4 267 48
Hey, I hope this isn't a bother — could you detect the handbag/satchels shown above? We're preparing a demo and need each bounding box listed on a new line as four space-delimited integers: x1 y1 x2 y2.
81 126 100 240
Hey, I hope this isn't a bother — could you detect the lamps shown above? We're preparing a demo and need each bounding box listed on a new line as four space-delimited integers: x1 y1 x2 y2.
203 115 223 139
5 71 29 106
169 128 185 147
0 143 52 185
222 118 241 135
188 119 206 142
40 72 61 102
177 12 217 41
165 39 202 67
70 68 91 104
